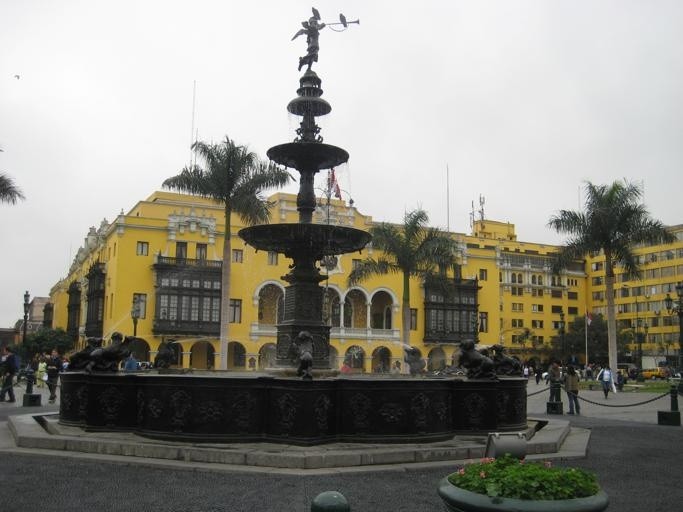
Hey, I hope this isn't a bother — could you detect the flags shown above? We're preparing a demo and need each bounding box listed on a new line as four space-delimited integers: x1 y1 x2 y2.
330 167 342 199
586 308 592 326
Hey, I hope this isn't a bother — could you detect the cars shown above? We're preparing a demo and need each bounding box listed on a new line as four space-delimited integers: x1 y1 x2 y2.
541 367 581 382
617 369 630 383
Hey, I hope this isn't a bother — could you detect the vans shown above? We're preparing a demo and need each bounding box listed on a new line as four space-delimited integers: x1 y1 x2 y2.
637 367 666 380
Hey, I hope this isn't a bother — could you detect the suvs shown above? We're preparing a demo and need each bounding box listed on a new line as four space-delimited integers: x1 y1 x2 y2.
618 363 639 380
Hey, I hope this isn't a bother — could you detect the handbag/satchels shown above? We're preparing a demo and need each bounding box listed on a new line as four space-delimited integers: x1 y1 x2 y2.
599 373 603 380
612 384 616 393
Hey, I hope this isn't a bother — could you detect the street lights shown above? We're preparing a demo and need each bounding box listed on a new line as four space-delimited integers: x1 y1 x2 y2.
22 289 30 342
664 281 683 395
313 185 354 328
558 308 565 361
131 298 141 337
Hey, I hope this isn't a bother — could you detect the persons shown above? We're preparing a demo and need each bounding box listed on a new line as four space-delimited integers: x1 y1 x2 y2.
0 344 153 405
391 360 401 374
340 361 352 372
524 364 673 415
292 8 325 71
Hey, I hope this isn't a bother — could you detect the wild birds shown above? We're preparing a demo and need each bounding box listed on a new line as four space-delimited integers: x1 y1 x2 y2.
340 13 348 27
312 7 321 20
